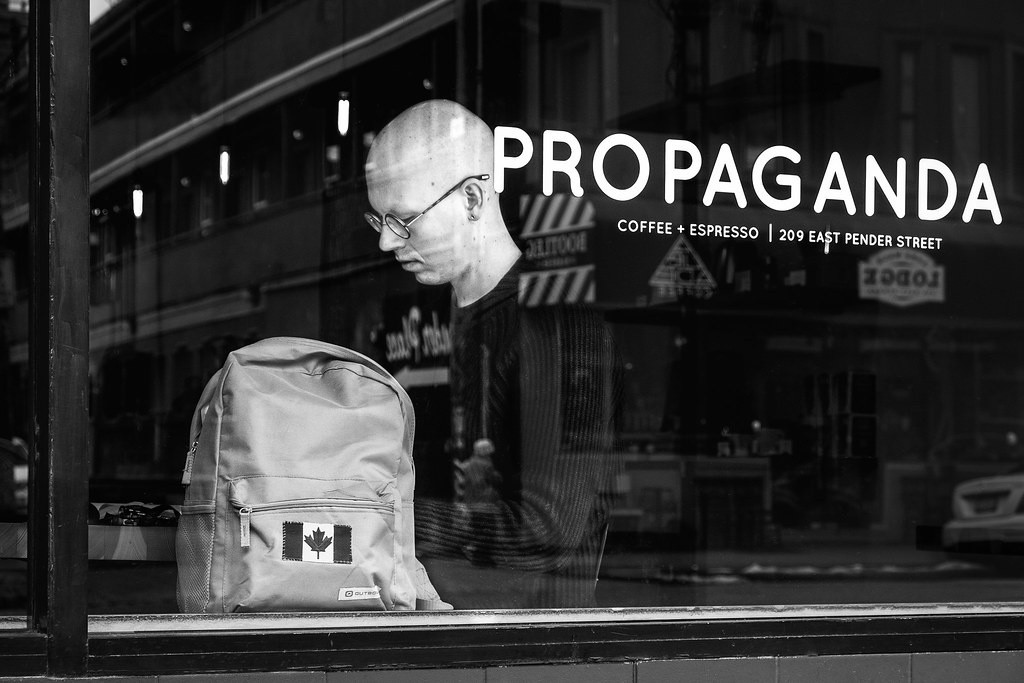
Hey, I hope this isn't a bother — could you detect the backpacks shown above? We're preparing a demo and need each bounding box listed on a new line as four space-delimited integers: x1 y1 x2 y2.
177 336 454 614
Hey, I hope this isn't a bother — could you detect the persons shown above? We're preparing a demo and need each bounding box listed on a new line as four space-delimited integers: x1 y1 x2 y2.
364 98 618 612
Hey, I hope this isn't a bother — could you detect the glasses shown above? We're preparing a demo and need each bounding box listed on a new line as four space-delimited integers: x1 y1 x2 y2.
363 174 490 239
